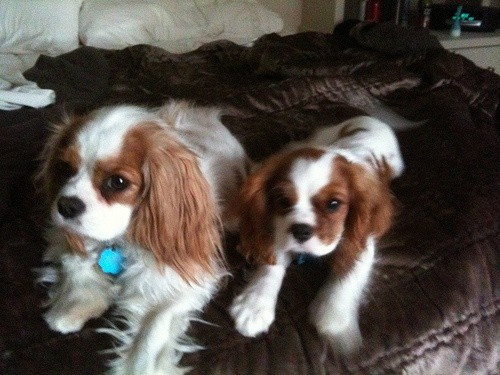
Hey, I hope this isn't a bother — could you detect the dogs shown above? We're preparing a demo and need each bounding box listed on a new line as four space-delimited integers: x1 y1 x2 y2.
216 114 406 356
26 94 253 375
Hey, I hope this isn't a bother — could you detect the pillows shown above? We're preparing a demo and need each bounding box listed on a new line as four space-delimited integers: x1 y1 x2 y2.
0 0 79 56
78 0 285 54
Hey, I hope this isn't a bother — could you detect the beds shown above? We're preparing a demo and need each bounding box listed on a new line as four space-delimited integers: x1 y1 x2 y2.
0 19 500 375
